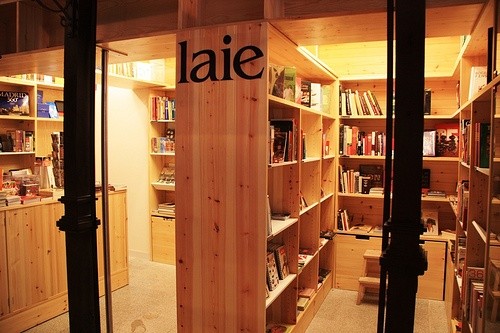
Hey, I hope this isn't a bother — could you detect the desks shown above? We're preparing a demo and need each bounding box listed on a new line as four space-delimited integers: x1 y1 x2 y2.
363 249 380 277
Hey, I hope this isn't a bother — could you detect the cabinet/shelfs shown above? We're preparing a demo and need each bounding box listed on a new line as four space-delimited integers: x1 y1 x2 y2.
175 26 337 333
148 88 175 265
338 1 500 333
0 77 129 333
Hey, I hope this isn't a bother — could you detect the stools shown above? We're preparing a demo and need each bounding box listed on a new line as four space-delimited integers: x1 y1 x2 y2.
356 277 388 305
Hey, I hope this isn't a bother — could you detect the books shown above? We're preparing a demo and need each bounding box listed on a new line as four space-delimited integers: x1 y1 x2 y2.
339 85 383 116
0 182 53 206
37 100 64 118
338 164 385 195
450 66 500 333
268 118 329 165
157 167 175 184
151 128 175 153
36 132 64 188
265 229 337 333
337 207 382 233
158 202 176 216
421 80 460 238
267 187 327 235
0 130 34 152
268 63 322 111
151 96 176 120
339 124 386 156
